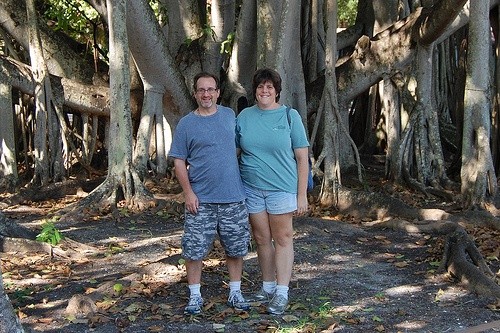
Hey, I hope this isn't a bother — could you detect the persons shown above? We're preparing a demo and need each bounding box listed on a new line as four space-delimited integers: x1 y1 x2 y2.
236 67 310 314
168 74 251 314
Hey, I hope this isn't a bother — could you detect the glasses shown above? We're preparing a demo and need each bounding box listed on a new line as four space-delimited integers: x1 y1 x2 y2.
196 87 218 93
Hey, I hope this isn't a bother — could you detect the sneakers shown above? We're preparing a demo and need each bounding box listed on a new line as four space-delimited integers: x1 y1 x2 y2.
246 289 275 302
184 294 203 313
266 294 289 314
227 290 250 312
